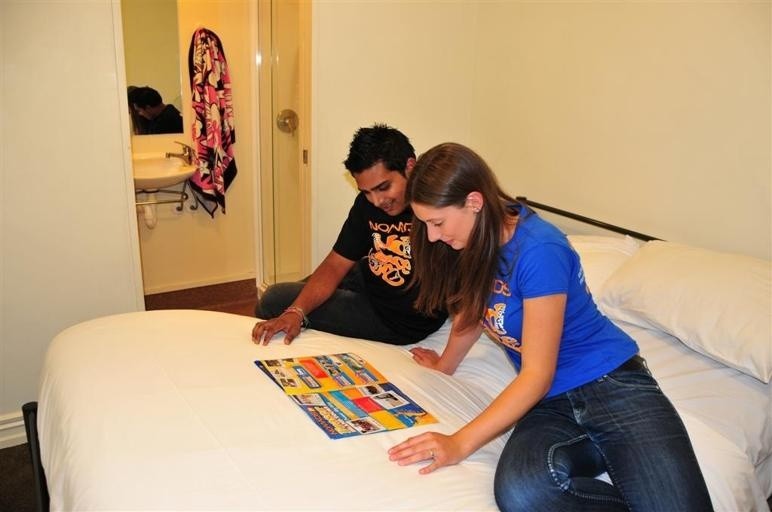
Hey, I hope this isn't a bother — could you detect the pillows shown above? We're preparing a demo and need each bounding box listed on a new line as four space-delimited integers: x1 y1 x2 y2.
567 234 657 329
600 240 772 384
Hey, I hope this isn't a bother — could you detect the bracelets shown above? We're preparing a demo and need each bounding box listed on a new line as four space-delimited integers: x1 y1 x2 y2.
282 306 308 331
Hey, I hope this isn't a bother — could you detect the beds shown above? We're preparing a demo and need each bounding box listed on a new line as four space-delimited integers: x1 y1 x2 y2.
21 201 772 512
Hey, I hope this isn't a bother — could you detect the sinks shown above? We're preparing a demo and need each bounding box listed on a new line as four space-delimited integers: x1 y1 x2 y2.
132 152 199 188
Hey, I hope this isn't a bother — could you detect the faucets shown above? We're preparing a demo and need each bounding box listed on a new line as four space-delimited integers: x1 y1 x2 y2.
165 141 196 165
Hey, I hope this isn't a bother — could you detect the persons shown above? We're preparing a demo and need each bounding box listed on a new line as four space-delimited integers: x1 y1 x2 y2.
134 88 183 135
385 140 717 512
127 85 151 135
250 122 449 347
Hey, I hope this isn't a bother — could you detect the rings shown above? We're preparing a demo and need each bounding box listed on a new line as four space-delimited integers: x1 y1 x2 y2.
429 449 435 458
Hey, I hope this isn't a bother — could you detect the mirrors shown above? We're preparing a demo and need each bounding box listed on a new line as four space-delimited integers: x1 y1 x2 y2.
121 0 184 135
